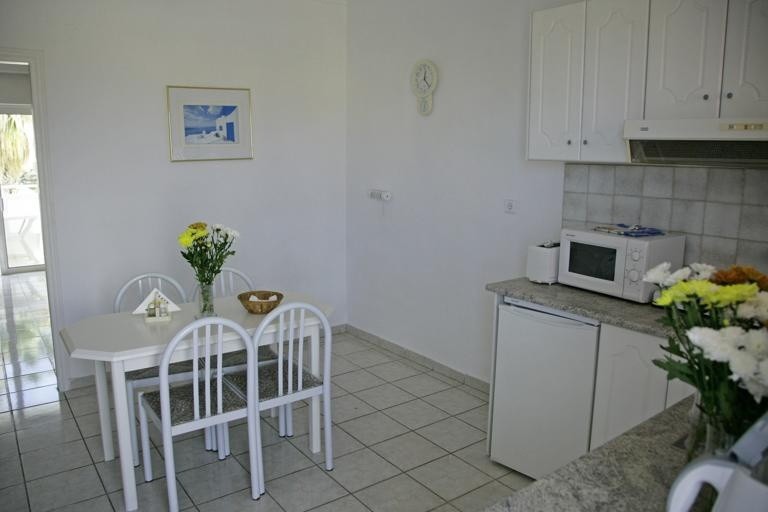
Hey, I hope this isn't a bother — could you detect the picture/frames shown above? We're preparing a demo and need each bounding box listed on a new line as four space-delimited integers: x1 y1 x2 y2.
166 85 254 163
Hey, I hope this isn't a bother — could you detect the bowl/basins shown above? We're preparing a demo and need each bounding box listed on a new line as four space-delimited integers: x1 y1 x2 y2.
237 291 284 315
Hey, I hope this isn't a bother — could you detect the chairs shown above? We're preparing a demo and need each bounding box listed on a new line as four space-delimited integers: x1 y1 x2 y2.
115 269 336 512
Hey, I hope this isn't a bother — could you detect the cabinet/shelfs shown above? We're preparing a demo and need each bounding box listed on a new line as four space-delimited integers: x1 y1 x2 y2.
487 295 704 481
527 0 768 161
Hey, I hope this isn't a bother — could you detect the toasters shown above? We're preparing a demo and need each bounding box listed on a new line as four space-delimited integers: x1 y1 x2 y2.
527 240 559 286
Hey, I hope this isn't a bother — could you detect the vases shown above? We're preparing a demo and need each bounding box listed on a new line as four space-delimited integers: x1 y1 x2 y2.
685 390 734 460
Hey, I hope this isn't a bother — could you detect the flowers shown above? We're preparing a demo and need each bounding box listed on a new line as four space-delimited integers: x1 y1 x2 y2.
643 260 768 451
178 222 240 310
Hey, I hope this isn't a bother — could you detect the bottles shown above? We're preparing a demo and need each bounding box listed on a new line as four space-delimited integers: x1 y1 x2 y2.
148 303 167 317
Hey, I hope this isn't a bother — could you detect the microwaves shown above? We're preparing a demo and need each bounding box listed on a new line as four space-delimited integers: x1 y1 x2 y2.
557 229 687 305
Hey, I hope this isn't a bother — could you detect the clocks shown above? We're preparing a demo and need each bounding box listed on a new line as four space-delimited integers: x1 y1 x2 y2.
409 60 438 115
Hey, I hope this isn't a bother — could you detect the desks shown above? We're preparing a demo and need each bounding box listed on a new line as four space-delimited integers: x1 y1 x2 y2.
59 293 335 512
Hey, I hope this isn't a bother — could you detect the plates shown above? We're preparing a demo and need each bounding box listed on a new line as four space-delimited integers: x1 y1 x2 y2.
145 312 170 323
